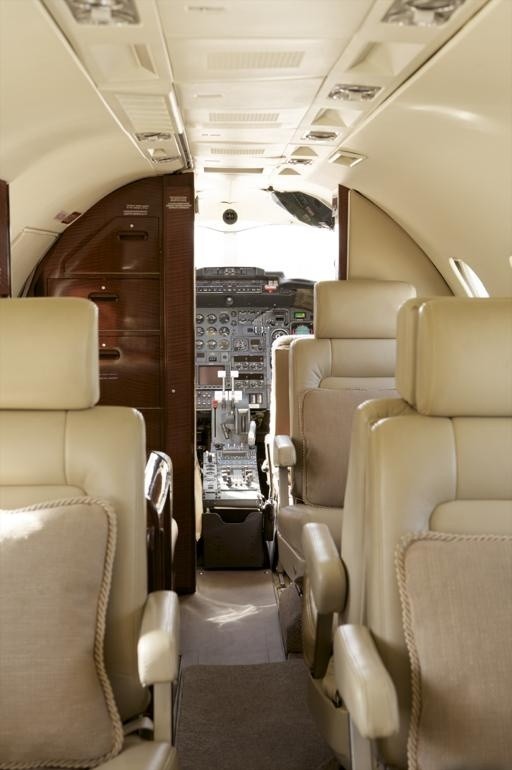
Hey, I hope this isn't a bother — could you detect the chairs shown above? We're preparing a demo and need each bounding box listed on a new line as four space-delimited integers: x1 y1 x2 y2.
300 290 468 758
334 294 512 770
0 295 181 762
264 276 426 657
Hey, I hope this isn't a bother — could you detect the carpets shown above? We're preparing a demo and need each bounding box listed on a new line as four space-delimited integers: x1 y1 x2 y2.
177 660 336 763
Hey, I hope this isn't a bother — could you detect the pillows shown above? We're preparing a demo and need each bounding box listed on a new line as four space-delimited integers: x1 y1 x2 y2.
0 497 123 763
392 523 512 770
